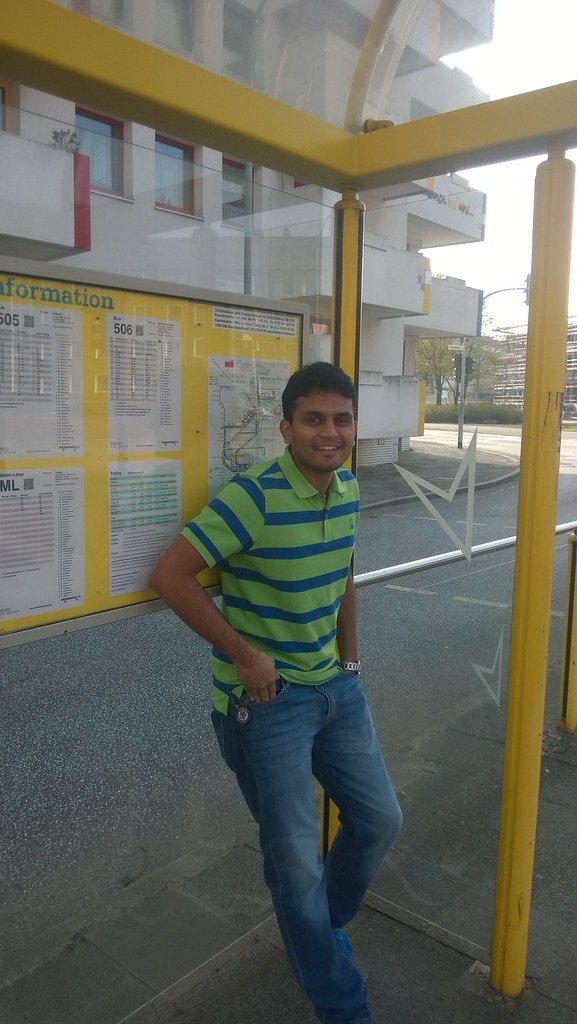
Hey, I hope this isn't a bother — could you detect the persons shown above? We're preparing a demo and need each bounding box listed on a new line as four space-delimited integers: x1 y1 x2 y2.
148 362 403 1024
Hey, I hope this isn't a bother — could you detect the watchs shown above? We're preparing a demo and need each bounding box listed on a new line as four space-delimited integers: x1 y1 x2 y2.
339 661 361 675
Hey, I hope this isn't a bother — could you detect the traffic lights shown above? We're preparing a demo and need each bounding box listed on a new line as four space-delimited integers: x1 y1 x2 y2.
524 273 530 305
452 354 461 383
465 357 474 375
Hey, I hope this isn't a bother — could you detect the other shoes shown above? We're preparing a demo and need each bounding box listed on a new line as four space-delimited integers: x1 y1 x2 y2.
332 926 368 984
